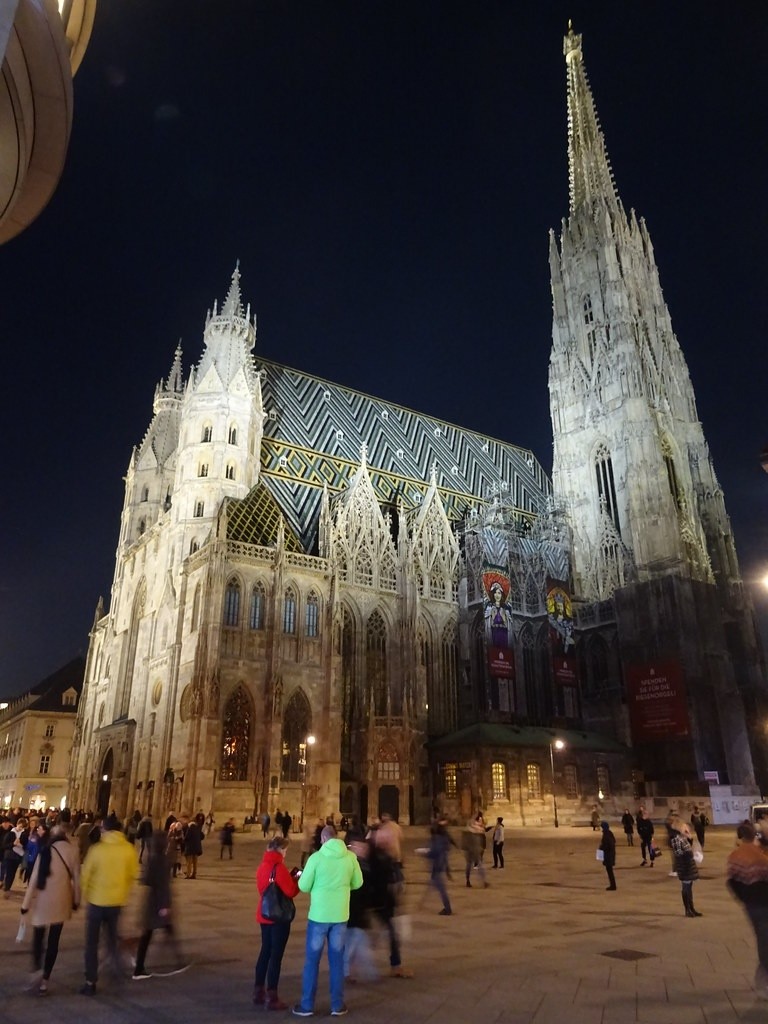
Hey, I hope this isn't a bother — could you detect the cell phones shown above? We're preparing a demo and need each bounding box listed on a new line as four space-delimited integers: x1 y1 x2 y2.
295 869 303 878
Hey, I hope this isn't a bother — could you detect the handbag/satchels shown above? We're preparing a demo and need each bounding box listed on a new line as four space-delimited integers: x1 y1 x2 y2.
649 838 662 857
260 863 296 921
595 850 604 861
690 837 703 866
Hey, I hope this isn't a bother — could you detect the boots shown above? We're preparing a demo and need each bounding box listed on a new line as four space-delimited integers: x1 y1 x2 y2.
253 985 268 1002
681 890 703 917
266 990 288 1010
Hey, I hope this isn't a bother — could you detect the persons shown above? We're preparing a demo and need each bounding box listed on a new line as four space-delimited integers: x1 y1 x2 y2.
80 817 138 992
263 808 292 839
599 821 617 890
622 807 654 868
220 818 235 858
591 808 599 831
251 837 301 1010
132 833 192 978
0 807 216 904
664 809 702 917
341 813 413 983
292 825 363 1017
691 806 710 847
491 817 504 869
726 822 768 971
300 814 338 868
420 817 458 915
20 824 80 995
462 812 495 888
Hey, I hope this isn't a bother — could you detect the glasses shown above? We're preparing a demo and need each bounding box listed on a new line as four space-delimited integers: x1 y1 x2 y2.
347 844 363 850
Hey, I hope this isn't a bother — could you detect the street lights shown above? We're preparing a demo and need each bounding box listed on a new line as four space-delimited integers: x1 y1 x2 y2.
550 740 564 827
299 735 315 832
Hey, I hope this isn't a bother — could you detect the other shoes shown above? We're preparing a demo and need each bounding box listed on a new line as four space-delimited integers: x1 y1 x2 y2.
650 863 654 868
492 865 498 869
668 871 679 876
292 1004 315 1016
139 858 143 864
465 882 473 888
483 881 490 889
332 1004 349 1015
499 866 504 869
606 886 616 890
392 965 415 978
25 945 196 997
440 908 453 915
640 860 647 866
168 867 197 879
